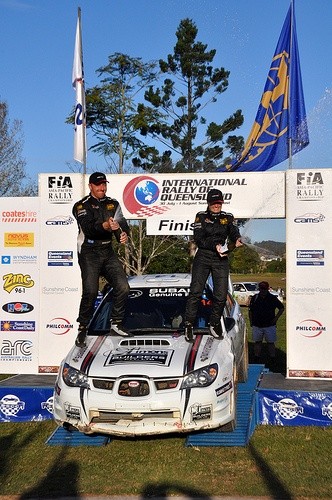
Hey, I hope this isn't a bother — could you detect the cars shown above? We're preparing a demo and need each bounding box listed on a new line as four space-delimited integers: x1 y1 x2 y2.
232 281 284 307
52 274 249 437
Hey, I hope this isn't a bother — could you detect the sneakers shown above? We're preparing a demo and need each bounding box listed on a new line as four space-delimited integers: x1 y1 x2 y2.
210 317 222 337
185 323 193 341
75 324 88 346
110 322 133 336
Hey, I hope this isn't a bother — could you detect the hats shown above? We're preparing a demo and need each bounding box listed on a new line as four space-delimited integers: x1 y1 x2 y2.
207 189 224 204
89 171 109 185
260 282 269 289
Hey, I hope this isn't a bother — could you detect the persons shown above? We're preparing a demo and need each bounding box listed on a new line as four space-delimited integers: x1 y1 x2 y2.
183 189 243 343
268 287 273 291
249 282 284 364
72 172 133 347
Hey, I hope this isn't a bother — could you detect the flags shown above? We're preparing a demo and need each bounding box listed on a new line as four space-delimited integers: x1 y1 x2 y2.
218 0 311 172
71 19 87 163
